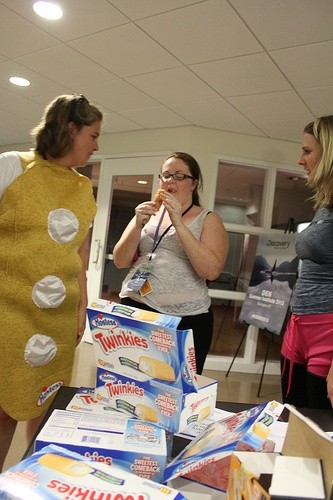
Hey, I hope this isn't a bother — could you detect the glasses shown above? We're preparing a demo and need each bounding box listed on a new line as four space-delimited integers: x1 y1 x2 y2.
158 172 195 181
71 94 89 121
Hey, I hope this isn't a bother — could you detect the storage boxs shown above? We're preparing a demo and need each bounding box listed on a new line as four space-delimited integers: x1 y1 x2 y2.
0 297 333 500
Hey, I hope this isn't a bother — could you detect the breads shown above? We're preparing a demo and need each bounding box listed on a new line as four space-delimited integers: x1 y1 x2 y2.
151 189 167 211
130 310 160 321
253 422 270 439
197 406 210 421
134 404 158 422
138 356 176 382
37 454 94 478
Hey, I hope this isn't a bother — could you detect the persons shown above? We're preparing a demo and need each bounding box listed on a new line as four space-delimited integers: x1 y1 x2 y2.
113 151 230 378
280 116 333 432
0 94 102 480
